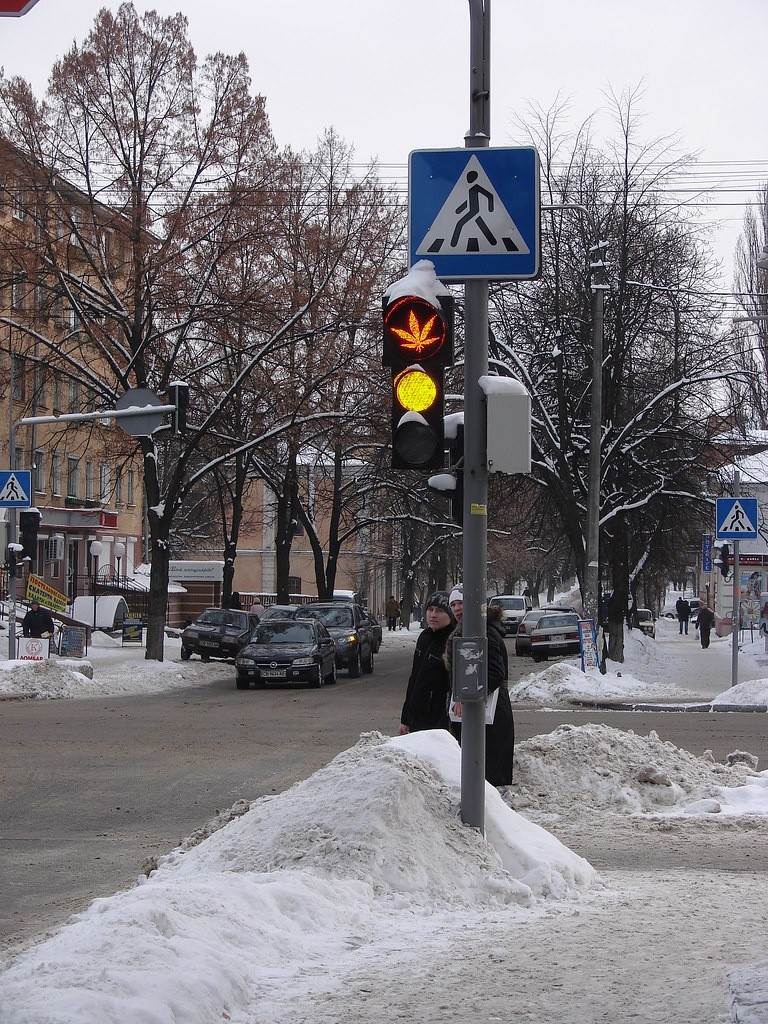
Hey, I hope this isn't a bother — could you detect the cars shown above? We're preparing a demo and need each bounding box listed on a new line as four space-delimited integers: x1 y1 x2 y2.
233 617 340 689
634 610 657 639
515 609 566 655
543 606 578 613
180 607 260 663
531 612 585 662
255 606 301 628
362 610 386 653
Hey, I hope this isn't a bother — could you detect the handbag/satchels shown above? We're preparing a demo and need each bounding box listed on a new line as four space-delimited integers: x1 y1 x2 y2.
396 611 400 617
694 627 699 640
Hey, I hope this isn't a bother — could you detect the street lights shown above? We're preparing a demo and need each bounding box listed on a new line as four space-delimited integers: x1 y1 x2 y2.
113 542 126 596
89 541 102 631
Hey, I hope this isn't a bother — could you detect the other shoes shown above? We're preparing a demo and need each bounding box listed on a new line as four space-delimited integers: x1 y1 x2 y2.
389 627 391 631
393 628 395 631
685 632 688 635
679 632 682 634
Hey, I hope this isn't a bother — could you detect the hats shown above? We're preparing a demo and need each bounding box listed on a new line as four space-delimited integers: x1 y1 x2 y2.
425 591 454 621
449 582 463 605
702 606 707 609
254 597 260 602
31 598 39 605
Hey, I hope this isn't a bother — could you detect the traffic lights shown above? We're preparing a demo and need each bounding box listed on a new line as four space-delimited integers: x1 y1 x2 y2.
432 417 464 525
715 543 732 578
380 277 448 472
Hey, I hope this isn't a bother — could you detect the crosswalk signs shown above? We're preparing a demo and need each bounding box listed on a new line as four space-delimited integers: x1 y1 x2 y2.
715 497 761 542
0 470 32 510
407 145 542 285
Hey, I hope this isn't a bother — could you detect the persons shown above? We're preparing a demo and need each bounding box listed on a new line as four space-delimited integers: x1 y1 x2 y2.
225 591 243 610
628 597 632 610
399 599 413 630
695 603 715 649
397 591 458 736
250 597 265 616
443 583 515 787
679 600 692 635
521 586 531 601
386 596 400 631
676 597 683 621
23 600 55 639
178 616 192 629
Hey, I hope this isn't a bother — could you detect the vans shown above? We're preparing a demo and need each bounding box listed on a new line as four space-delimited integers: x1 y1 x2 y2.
489 594 534 638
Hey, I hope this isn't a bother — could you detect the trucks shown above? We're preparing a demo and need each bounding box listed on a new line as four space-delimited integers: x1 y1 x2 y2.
660 591 700 620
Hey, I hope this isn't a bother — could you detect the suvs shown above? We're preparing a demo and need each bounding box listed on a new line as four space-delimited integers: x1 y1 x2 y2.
292 599 376 678
